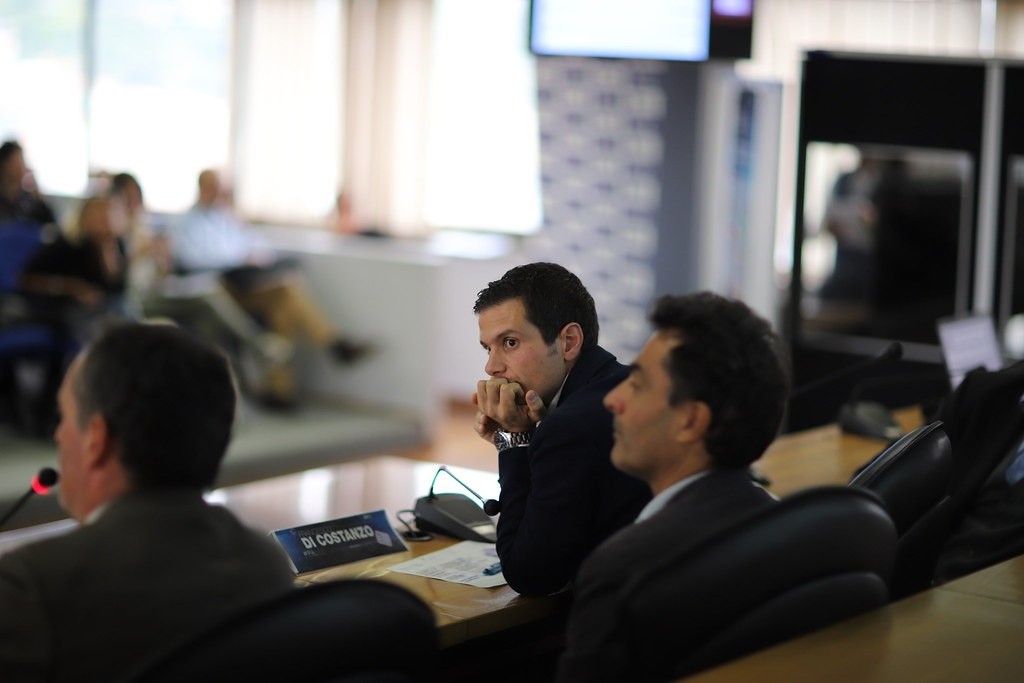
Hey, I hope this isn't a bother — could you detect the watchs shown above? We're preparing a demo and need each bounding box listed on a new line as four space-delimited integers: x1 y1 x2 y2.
494 428 533 453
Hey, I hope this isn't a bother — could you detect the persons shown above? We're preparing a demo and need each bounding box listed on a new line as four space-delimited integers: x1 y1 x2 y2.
0 323 295 682
818 144 932 341
0 140 379 417
553 290 787 683
472 263 653 599
941 363 1024 513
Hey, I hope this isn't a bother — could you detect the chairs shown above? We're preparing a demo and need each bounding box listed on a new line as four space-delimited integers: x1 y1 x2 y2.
573 484 902 683
843 419 953 598
124 578 445 683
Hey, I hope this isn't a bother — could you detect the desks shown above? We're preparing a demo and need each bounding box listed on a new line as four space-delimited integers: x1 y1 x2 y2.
742 404 940 496
0 453 519 683
696 561 1024 683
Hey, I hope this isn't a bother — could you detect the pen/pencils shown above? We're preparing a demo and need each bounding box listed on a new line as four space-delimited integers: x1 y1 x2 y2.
482 561 501 575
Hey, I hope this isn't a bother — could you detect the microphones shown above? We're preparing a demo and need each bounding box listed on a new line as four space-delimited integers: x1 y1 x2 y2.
803 341 902 394
414 468 501 543
0 466 58 529
